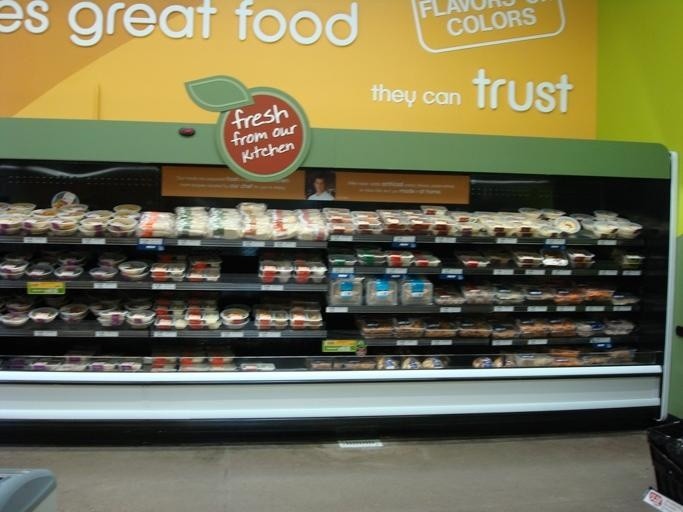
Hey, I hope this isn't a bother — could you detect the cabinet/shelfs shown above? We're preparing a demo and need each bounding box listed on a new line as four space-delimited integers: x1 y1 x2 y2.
0 235 662 424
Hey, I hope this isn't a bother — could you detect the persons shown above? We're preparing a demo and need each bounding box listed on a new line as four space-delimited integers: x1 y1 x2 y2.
306 175 334 201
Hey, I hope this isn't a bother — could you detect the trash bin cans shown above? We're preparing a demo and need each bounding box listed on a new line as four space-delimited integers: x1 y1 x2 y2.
647 419 683 507
0 468 57 512
